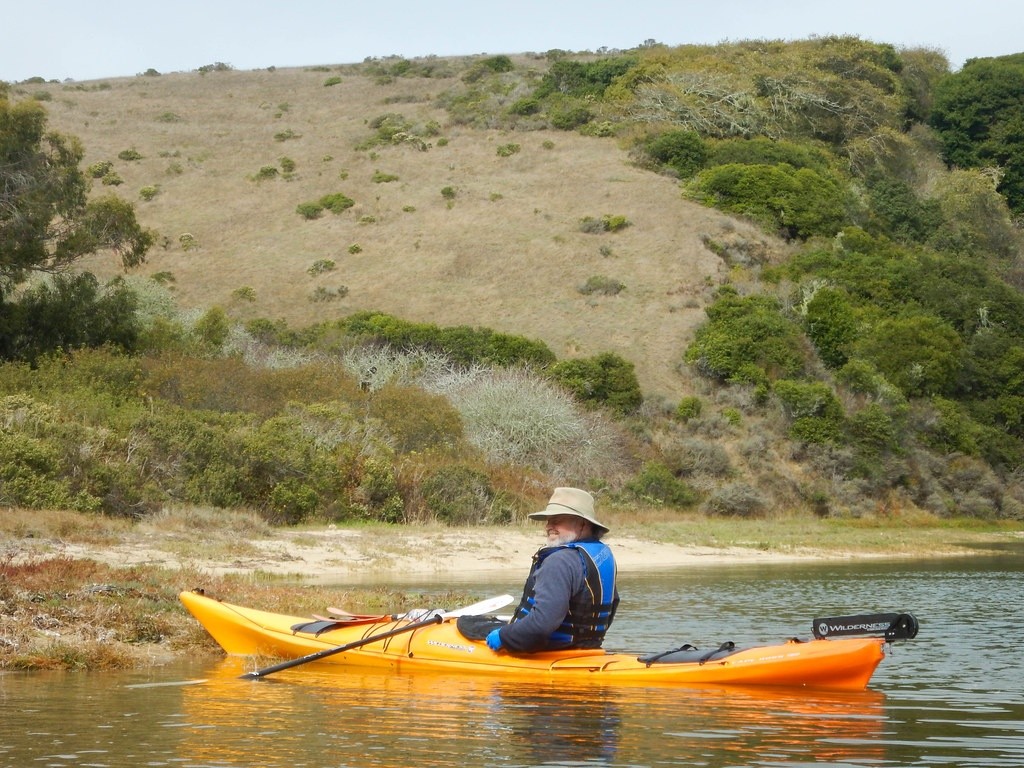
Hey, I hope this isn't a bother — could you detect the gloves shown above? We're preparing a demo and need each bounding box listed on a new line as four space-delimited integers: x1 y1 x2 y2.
486 626 503 651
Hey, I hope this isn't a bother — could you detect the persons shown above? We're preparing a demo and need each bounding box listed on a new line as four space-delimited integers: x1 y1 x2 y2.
485 486 620 652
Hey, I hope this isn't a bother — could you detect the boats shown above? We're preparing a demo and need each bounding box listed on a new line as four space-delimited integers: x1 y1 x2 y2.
177 590 919 691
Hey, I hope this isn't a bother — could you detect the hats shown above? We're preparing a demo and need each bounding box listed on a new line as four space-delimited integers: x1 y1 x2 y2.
528 486 608 538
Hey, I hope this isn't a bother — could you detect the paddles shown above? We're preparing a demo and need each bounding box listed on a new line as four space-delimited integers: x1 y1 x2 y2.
238 593 516 680
312 606 516 626
